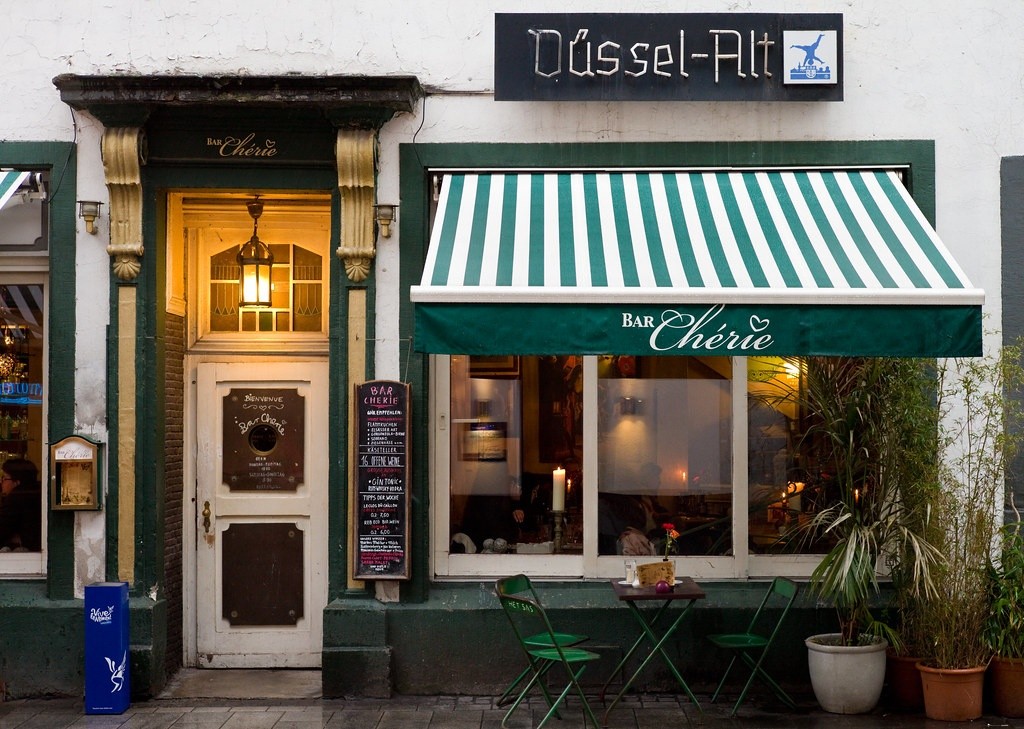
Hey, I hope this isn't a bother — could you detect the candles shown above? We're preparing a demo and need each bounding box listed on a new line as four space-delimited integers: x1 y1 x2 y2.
682 472 686 482
782 492 786 503
552 466 566 513
567 479 572 494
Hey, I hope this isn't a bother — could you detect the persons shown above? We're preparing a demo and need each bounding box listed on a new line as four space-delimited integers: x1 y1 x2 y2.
445 473 687 557
0 458 41 555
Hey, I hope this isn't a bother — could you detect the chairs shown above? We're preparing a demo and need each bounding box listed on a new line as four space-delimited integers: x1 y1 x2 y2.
705 576 800 718
495 573 601 729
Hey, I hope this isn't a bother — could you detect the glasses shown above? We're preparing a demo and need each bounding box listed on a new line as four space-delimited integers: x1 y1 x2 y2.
1 476 15 483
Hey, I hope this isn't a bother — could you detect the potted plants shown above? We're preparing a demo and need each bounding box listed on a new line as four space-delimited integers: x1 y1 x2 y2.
746 312 1024 722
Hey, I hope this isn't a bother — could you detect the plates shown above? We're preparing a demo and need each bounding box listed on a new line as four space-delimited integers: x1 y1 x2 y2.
619 581 639 586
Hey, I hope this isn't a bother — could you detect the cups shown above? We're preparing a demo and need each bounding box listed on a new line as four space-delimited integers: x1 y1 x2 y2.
624 559 636 582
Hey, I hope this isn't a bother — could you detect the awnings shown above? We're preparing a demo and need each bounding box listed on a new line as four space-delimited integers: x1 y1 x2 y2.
0 172 47 212
408 175 986 357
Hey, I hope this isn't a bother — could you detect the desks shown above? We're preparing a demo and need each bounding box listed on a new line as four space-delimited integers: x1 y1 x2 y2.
600 576 706 729
748 509 812 546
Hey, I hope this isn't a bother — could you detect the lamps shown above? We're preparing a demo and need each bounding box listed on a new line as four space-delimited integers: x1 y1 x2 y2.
75 199 105 233
235 201 274 308
372 203 400 238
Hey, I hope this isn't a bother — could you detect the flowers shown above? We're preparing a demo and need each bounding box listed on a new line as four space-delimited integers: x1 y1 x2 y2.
661 523 681 560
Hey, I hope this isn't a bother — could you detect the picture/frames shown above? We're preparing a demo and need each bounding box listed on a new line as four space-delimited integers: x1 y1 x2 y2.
470 355 520 378
538 355 642 462
